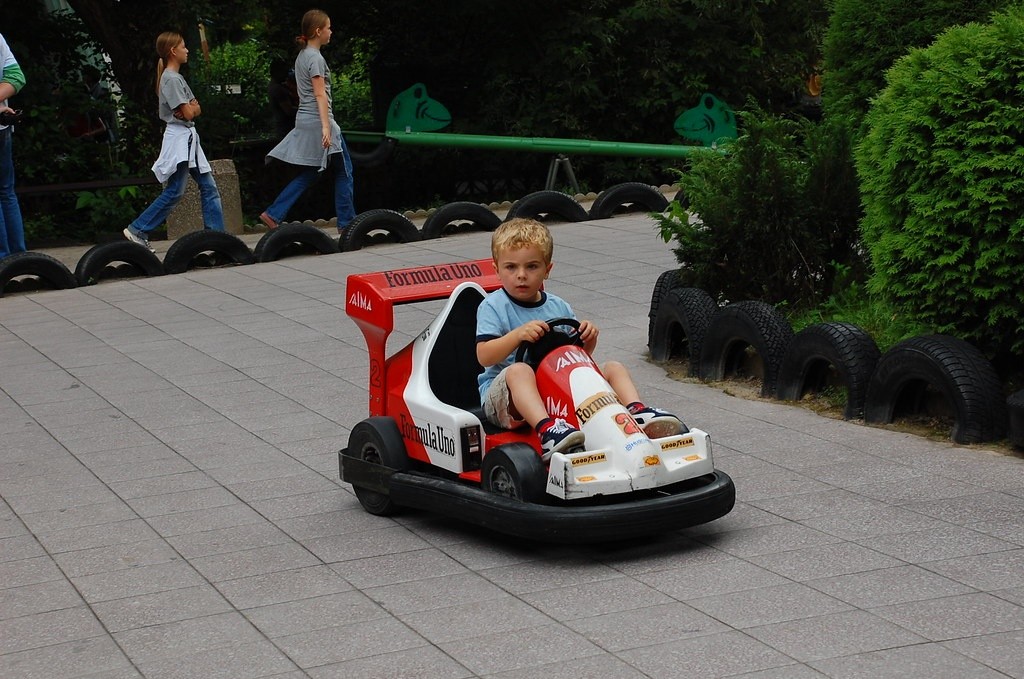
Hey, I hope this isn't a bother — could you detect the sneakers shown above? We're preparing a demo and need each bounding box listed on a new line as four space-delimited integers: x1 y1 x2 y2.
260 212 278 230
540 417 586 462
124 229 156 253
337 227 344 233
629 406 690 438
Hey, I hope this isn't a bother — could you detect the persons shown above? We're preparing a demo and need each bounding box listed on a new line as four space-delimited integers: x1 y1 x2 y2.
259 9 357 234
475 217 680 462
123 32 225 253
0 32 27 258
